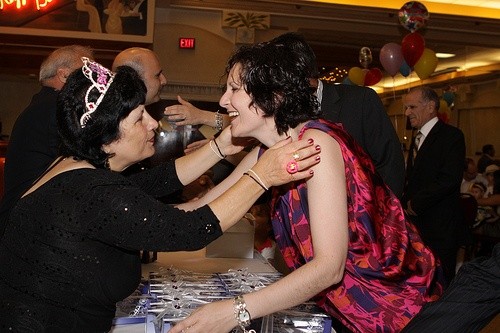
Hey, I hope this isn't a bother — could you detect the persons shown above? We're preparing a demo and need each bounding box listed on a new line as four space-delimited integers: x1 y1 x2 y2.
403 86 465 294
0 46 93 212
402 243 500 333
0 57 320 333
113 48 166 105
460 144 500 229
267 31 403 199
169 44 444 332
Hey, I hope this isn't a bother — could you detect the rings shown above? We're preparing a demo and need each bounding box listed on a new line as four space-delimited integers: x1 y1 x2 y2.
185 114 186 119
293 153 299 161
287 160 298 173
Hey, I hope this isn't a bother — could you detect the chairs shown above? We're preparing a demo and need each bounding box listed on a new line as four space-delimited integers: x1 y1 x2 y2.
461 193 478 254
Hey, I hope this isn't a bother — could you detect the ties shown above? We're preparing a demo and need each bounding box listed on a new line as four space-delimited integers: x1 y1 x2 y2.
411 131 422 168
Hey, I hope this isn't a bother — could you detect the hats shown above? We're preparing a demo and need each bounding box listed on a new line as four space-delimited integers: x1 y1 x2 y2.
485 165 500 175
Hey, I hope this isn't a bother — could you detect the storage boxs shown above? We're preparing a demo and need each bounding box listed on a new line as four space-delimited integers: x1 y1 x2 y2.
205 212 256 259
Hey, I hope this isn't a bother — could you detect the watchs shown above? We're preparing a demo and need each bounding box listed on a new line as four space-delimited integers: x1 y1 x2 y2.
234 296 252 330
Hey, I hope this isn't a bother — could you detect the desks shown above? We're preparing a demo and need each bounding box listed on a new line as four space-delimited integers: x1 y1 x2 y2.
108 246 336 333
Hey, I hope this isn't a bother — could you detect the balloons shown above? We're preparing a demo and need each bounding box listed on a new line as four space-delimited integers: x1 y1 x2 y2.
350 67 382 86
402 32 425 68
399 60 413 79
398 1 428 33
414 48 437 79
380 43 402 78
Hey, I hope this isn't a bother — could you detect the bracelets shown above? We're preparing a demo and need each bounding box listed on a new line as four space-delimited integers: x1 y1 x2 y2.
243 169 268 191
209 139 225 159
215 113 223 130
